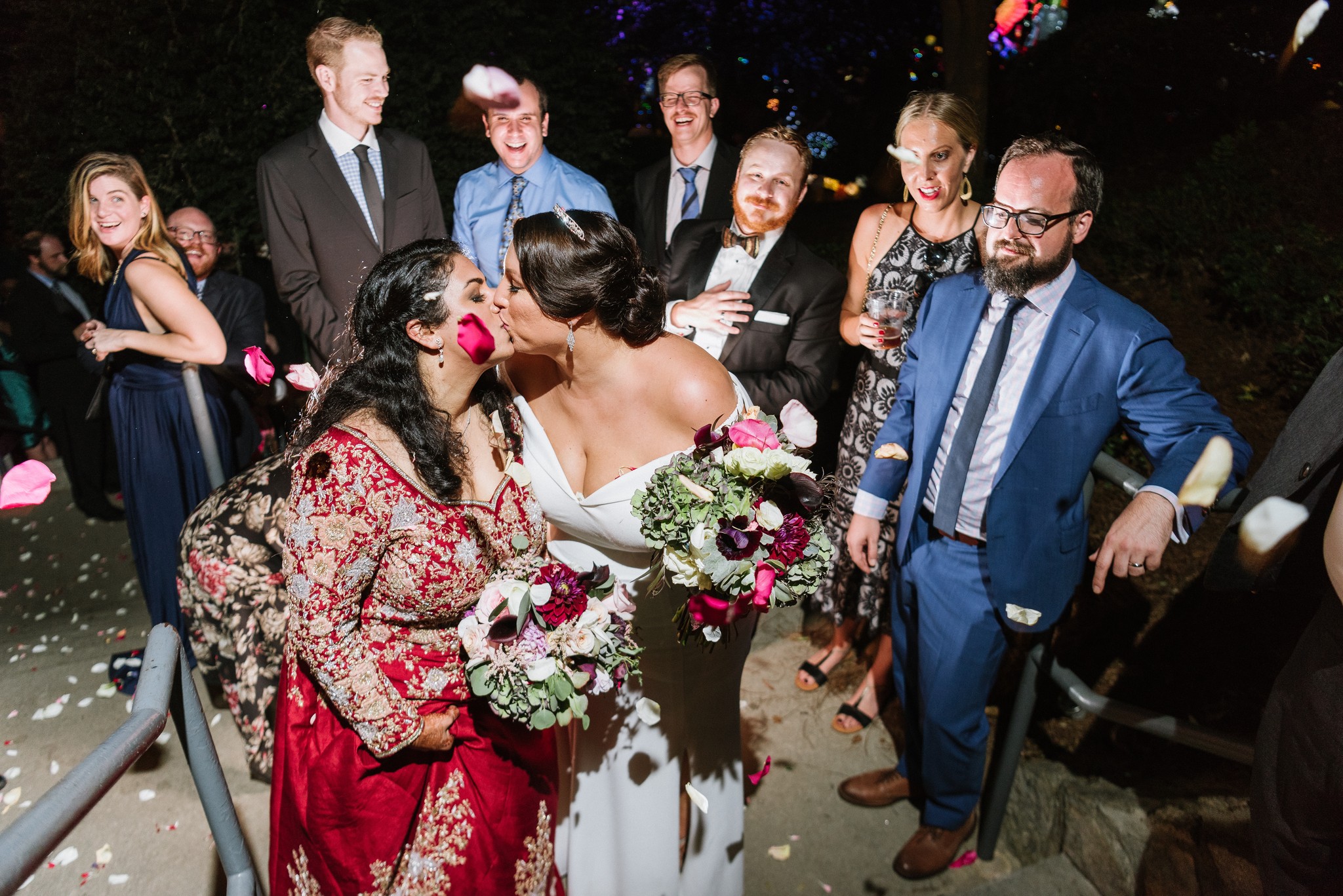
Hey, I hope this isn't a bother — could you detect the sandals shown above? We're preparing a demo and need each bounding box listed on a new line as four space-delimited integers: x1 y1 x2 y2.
830 679 894 733
795 638 857 691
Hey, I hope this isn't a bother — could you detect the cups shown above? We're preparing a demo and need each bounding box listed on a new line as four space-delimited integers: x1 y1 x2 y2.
864 289 910 348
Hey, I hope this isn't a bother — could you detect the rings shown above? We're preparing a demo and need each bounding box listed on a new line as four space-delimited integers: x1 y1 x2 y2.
91 348 96 355
720 311 725 321
1129 562 1143 568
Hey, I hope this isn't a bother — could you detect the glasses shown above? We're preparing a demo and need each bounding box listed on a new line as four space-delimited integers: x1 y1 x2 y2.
658 91 714 108
981 202 1085 235
166 225 220 245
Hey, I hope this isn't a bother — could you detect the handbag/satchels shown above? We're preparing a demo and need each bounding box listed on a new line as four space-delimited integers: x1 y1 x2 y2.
84 361 115 421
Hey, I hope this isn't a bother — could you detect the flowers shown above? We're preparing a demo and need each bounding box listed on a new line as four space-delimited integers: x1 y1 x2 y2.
628 399 847 657
452 530 647 737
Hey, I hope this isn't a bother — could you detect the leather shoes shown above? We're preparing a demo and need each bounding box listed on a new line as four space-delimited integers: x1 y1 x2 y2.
837 767 910 808
893 805 977 879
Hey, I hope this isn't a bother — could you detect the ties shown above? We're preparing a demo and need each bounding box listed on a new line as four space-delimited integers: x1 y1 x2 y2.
932 294 1031 537
496 176 530 282
352 144 386 253
677 165 703 221
196 285 204 302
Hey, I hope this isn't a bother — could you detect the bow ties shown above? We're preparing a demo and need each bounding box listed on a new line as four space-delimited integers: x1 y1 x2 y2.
721 225 759 259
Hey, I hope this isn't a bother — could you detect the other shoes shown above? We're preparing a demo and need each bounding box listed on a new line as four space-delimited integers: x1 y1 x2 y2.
92 504 126 523
104 480 123 493
25 435 58 462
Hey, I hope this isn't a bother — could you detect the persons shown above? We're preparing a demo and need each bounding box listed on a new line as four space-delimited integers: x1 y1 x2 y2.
489 204 790 896
161 205 267 471
620 52 745 283
251 15 452 380
11 230 131 524
651 122 850 425
177 232 576 896
0 344 60 464
452 70 622 290
791 90 995 736
63 151 235 709
835 132 1254 882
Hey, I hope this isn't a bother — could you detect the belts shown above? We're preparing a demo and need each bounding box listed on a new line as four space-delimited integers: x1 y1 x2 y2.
917 504 988 549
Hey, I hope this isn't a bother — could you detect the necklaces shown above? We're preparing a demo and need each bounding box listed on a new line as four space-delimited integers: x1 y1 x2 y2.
448 394 472 458
